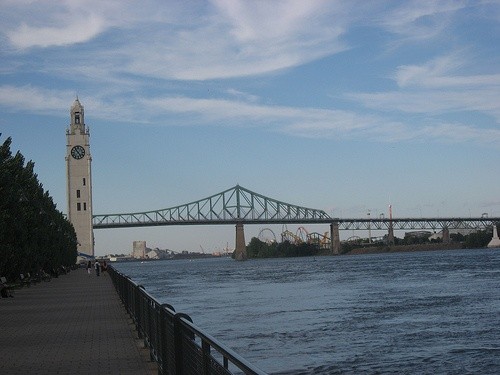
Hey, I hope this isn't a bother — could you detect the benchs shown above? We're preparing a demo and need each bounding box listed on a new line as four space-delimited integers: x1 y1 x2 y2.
1 263 85 293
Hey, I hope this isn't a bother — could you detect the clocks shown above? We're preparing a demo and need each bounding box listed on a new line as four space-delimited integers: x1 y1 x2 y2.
70 145 85 159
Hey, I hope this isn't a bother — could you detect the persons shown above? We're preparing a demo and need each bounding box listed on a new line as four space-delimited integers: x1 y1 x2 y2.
103 258 108 277
40 261 88 279
94 260 101 277
88 261 92 279
